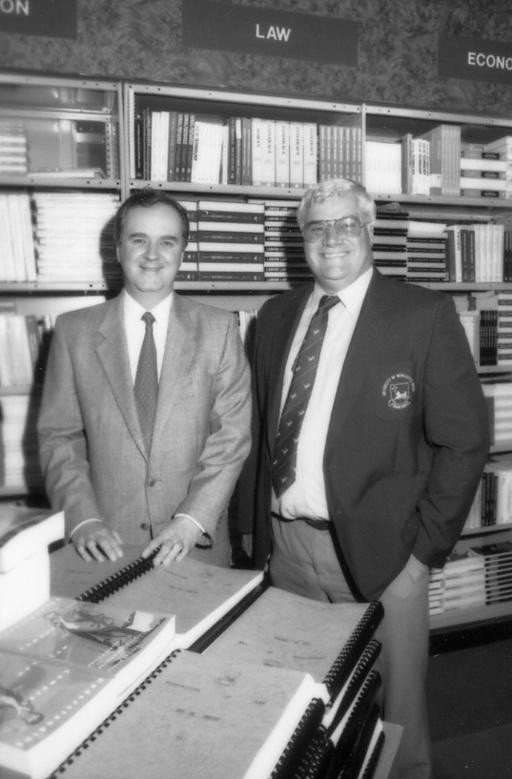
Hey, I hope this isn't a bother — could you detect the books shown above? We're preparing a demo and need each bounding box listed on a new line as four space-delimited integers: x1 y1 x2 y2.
426 542 512 612
132 106 508 201
169 201 316 283
468 469 512 529
368 213 512 368
0 83 120 490
0 499 386 779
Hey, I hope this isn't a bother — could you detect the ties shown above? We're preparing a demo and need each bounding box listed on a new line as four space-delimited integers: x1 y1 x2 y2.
134 313 158 459
272 296 340 497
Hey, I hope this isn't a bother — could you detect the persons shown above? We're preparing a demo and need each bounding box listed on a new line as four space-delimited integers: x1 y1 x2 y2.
230 176 488 779
33 191 253 569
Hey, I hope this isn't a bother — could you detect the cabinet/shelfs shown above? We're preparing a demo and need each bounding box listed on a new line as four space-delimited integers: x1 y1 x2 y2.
124 81 366 353
0 73 127 514
365 102 512 654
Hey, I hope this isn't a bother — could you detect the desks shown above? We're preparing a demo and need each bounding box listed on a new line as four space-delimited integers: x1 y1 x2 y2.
374 721 405 779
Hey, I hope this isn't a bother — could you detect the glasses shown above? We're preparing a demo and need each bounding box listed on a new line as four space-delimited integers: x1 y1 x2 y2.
303 218 366 237
